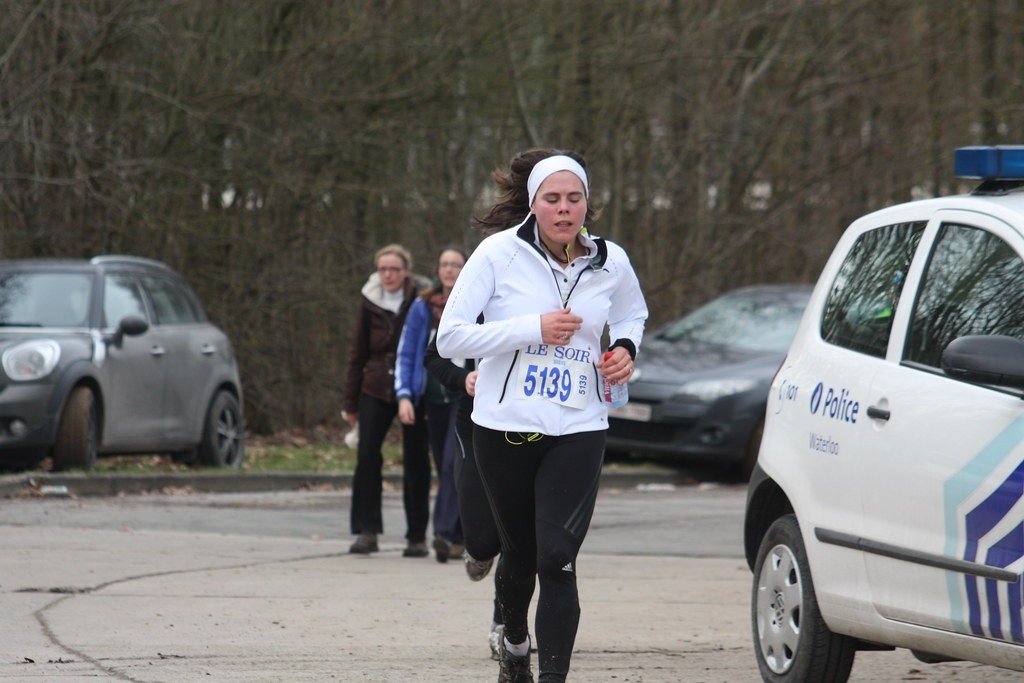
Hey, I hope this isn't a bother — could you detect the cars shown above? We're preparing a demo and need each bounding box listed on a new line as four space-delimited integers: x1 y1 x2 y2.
0 254 247 475
740 144 1024 683
600 282 903 479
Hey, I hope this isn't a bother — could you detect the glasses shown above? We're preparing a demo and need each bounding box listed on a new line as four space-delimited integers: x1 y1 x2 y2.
376 267 404 273
439 262 462 269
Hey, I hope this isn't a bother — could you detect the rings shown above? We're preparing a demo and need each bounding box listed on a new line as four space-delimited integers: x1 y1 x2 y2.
562 331 567 339
626 365 631 371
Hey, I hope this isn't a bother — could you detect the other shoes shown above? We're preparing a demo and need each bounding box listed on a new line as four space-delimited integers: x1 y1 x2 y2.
402 539 429 557
448 543 465 559
349 533 379 554
432 536 453 564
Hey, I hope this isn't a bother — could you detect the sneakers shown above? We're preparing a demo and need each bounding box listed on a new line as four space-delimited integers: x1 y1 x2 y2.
462 547 494 582
488 618 505 661
498 630 534 683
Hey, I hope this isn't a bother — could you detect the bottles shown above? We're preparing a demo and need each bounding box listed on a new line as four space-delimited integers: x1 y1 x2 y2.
602 352 629 408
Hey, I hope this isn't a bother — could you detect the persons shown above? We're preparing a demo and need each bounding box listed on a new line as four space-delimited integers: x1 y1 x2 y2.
436 148 648 683
393 245 504 660
342 243 430 557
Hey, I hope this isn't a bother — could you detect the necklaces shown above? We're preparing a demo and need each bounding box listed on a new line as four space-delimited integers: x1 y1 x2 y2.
539 237 573 263
